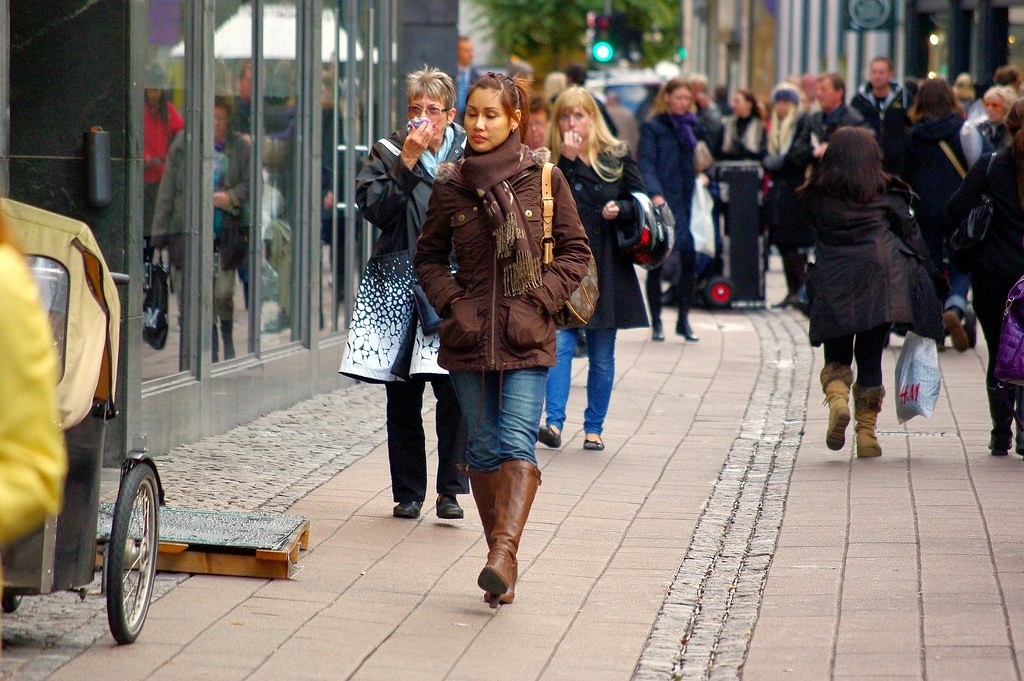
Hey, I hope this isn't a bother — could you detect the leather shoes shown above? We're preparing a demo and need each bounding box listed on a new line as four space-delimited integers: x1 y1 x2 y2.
652 325 664 341
393 501 423 518
676 320 698 341
436 494 463 518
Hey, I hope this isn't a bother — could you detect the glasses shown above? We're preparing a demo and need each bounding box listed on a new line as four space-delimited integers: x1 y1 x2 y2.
407 104 450 116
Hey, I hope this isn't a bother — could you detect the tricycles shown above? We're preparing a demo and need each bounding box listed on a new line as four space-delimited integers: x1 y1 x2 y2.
0 199 163 646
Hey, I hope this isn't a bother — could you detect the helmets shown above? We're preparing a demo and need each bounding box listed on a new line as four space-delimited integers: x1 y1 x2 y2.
616 192 676 271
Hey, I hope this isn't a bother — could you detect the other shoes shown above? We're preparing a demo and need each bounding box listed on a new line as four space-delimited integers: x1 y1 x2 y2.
943 311 969 352
265 314 291 333
583 438 605 450
538 427 561 448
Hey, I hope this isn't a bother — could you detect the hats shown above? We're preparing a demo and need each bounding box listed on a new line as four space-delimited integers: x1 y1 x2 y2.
771 82 800 106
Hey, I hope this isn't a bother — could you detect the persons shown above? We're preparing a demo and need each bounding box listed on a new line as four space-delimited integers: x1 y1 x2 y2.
413 72 591 602
0 240 68 554
142 60 367 363
339 64 470 520
456 34 1024 353
943 99 1024 456
536 85 648 450
776 127 922 458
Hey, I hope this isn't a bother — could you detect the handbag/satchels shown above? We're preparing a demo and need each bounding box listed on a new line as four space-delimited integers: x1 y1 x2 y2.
895 331 941 424
410 281 444 336
535 162 601 329
994 276 1024 431
142 246 174 350
946 152 997 273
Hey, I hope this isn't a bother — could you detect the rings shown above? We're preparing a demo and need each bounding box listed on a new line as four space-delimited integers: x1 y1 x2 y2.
426 137 428 141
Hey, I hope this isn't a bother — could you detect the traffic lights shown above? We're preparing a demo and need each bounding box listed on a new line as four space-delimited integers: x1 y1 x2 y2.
591 14 615 65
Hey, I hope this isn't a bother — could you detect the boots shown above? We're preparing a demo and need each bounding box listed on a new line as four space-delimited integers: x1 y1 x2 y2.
456 461 542 608
852 382 886 457
820 362 854 451
